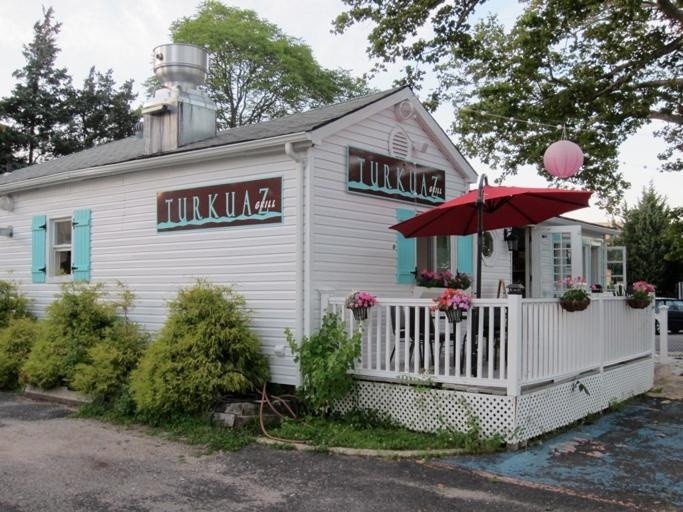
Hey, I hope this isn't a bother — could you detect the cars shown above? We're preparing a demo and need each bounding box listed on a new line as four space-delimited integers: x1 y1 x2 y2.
655 297 683 334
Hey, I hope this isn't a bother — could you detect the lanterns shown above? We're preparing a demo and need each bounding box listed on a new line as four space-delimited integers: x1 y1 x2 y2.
543 139 584 178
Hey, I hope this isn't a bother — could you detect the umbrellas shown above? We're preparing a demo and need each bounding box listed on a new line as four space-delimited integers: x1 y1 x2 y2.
388 173 595 358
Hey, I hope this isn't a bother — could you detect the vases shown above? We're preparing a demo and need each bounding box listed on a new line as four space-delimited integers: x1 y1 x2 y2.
560 301 591 312
625 300 651 309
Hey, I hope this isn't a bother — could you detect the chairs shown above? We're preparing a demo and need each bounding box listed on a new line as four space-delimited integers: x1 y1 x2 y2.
390 305 508 376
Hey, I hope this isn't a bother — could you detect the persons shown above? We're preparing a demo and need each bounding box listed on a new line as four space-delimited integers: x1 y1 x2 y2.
60 251 72 275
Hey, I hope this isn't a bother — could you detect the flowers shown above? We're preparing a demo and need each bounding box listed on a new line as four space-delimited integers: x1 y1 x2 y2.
427 288 476 316
556 272 596 300
417 268 475 289
344 290 377 309
622 280 657 304
